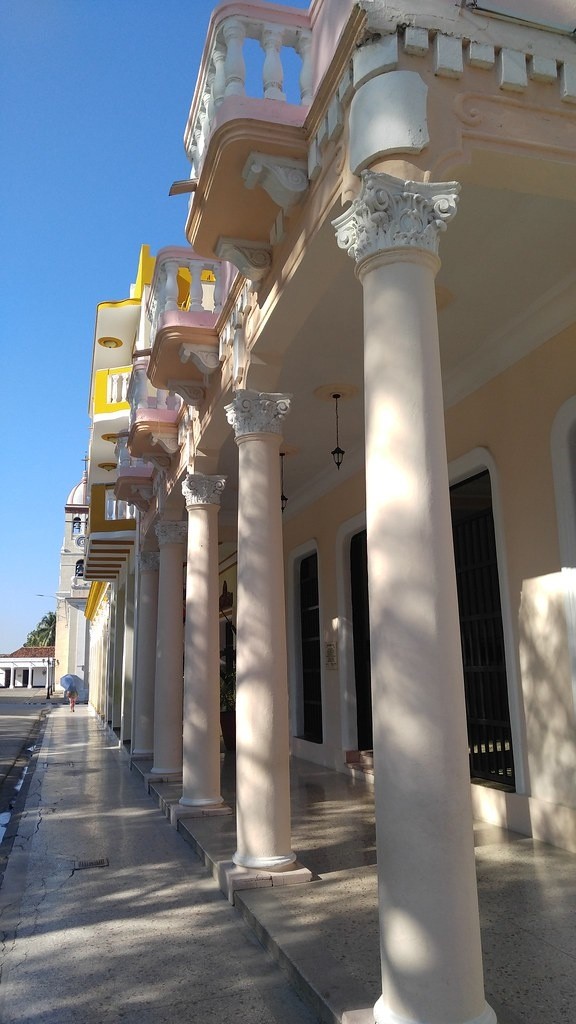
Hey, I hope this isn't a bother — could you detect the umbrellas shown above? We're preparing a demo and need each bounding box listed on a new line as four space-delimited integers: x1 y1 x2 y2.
60 674 83 691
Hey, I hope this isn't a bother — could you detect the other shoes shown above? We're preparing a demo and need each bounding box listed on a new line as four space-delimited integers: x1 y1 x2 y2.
70 709 74 713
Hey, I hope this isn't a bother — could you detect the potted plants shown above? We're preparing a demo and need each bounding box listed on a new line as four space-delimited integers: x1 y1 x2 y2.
220 670 235 752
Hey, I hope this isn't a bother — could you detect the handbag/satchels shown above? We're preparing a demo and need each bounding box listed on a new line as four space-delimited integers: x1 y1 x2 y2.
66 693 70 698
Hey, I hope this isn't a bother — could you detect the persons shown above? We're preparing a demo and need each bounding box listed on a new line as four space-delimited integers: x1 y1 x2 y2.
66 690 78 712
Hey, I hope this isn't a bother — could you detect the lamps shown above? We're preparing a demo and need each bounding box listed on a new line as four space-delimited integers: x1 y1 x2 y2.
279 453 288 513
331 394 345 470
